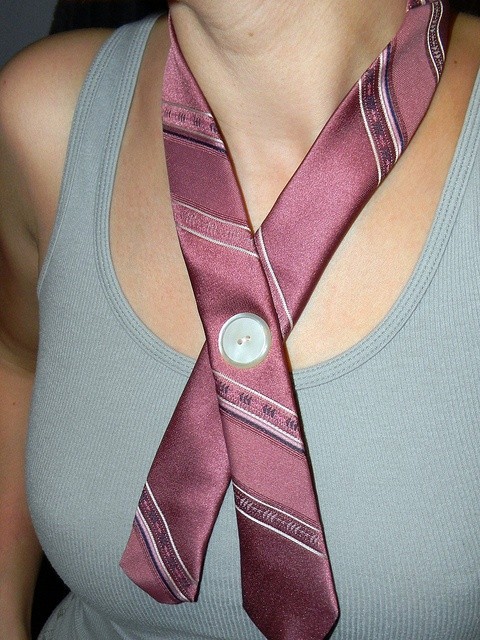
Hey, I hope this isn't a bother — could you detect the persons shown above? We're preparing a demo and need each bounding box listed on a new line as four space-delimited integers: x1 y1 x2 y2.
0 1 479 640
